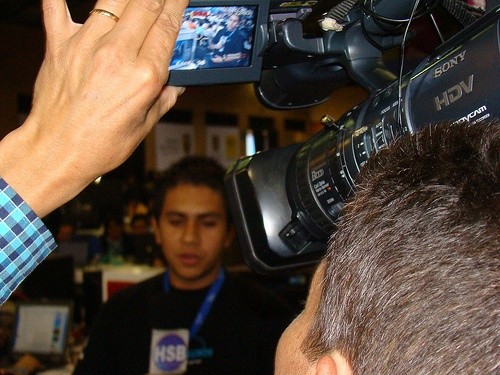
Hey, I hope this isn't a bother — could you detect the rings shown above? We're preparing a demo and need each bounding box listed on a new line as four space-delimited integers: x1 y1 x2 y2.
89 9 120 22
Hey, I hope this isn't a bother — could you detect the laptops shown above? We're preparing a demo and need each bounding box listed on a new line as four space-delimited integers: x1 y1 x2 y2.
10 302 68 370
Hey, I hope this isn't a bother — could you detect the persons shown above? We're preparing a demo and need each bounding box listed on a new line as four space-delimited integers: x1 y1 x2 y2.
170 7 256 70
0 0 500 375
0 153 291 375
